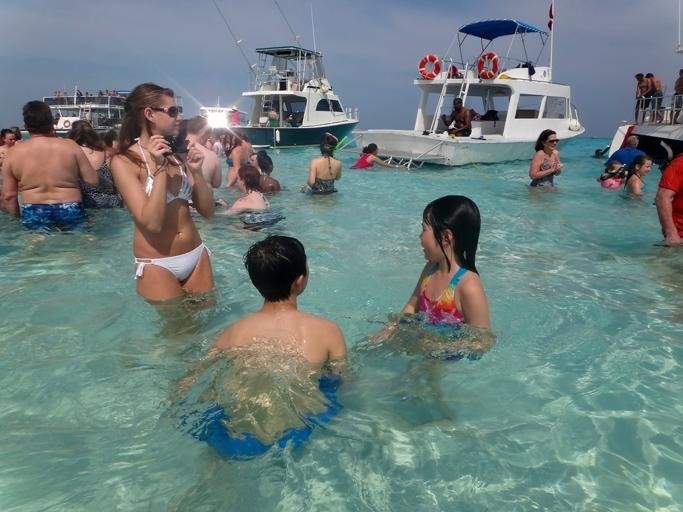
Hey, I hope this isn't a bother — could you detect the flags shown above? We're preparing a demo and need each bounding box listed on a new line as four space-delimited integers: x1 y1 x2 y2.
547 3 554 31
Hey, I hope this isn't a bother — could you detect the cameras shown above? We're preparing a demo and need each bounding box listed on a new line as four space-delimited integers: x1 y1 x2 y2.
159 135 191 156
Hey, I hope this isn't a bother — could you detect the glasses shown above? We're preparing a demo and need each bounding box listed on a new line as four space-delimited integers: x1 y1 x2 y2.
149 105 183 117
549 139 559 143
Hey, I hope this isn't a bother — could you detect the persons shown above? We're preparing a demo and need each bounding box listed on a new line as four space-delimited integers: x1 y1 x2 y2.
595 143 611 158
350 143 400 170
604 135 647 171
351 195 489 361
301 132 342 196
624 155 653 206
109 83 218 335
669 69 683 126
601 160 627 191
0 99 281 232
434 98 472 137
171 235 350 464
645 73 664 123
528 129 565 191
654 153 683 245
632 72 653 125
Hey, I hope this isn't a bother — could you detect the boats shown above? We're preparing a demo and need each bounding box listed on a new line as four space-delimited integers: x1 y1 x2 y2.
17 85 183 150
200 1 360 151
350 1 586 169
618 1 682 167
198 95 248 142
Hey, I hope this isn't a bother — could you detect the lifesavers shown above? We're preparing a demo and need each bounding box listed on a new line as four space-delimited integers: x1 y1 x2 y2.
419 54 440 80
478 52 500 79
